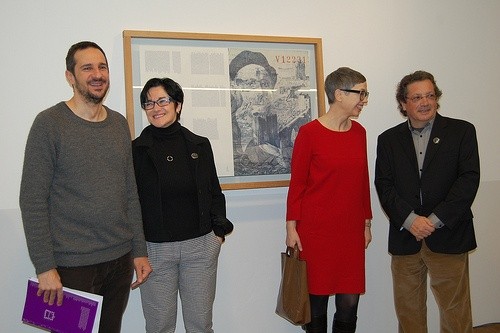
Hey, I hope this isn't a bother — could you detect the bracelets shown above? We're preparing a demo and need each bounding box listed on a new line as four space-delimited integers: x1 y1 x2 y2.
364 223 371 227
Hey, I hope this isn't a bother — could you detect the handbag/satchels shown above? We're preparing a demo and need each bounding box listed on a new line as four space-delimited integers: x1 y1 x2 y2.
275 244 311 326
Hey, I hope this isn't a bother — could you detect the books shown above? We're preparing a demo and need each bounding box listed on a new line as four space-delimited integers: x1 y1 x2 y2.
21 277 103 333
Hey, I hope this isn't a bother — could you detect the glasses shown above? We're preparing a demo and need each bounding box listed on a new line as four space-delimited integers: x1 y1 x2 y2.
145 96 176 110
340 88 369 100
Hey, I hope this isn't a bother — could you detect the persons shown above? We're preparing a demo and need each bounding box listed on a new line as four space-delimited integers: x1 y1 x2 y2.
374 71 481 333
19 41 153 333
286 67 372 333
131 76 233 333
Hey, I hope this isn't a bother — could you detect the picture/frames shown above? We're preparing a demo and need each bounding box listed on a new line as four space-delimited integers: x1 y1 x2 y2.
122 30 327 190
